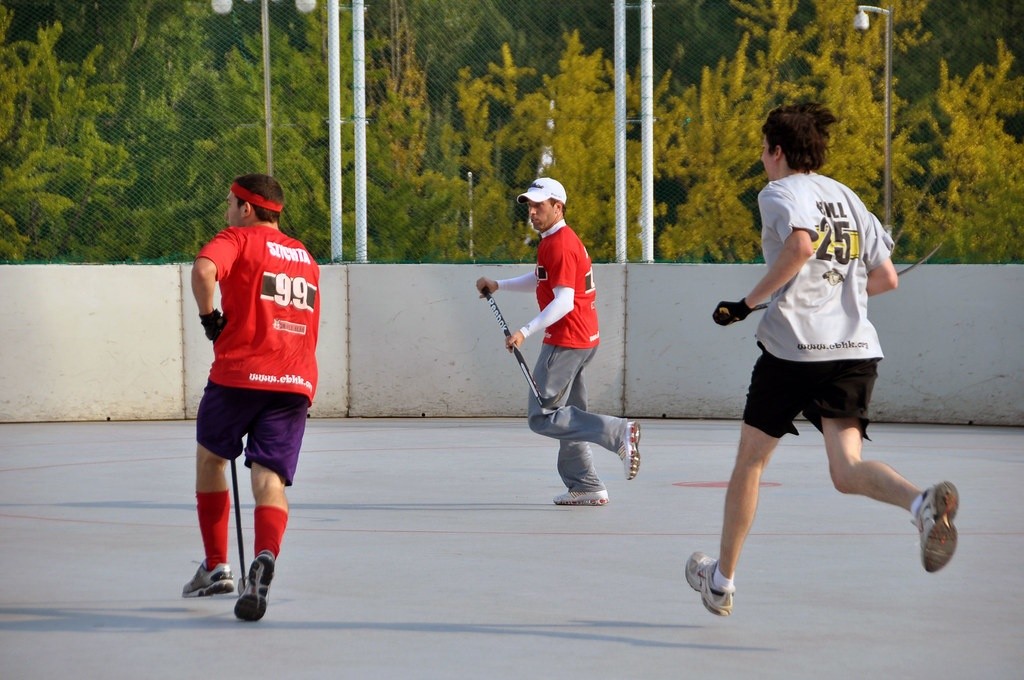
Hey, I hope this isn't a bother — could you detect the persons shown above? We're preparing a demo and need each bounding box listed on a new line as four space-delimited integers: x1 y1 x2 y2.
474 176 643 508
180 170 322 623
683 99 961 619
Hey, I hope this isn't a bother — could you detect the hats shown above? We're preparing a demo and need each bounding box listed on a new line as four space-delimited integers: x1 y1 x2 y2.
517 177 567 205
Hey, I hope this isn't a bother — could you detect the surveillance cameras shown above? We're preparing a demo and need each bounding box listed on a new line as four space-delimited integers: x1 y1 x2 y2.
854 13 869 32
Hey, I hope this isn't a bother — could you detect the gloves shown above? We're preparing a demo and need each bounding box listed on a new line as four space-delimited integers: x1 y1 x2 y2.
198 308 226 341
712 297 752 326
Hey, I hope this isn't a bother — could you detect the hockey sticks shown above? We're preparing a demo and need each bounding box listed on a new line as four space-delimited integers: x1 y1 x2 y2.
480 286 575 411
226 455 251 598
716 239 945 328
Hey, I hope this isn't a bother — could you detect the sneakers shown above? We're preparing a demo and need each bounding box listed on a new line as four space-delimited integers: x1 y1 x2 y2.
181 557 234 598
552 489 609 506
910 480 958 571
685 551 736 616
234 549 275 623
617 420 641 480
